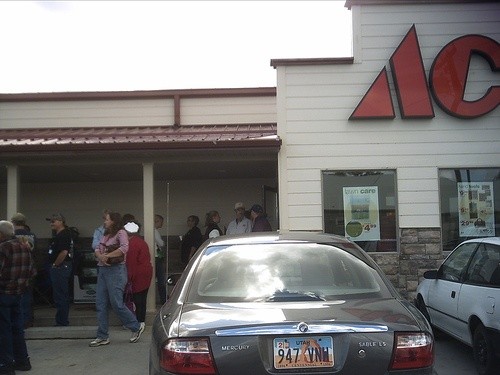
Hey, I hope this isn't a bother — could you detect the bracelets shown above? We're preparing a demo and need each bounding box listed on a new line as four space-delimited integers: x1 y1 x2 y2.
53 263 56 266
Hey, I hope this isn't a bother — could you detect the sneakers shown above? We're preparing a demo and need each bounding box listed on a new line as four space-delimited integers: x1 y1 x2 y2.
129 322 146 344
89 337 109 347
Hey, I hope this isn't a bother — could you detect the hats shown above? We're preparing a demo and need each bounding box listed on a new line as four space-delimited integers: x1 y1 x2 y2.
11 213 25 222
124 222 140 233
234 202 245 210
44 213 64 221
252 205 263 214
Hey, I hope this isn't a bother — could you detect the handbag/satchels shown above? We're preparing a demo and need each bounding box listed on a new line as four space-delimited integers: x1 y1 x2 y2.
157 246 167 259
106 243 124 264
124 281 135 315
54 260 73 276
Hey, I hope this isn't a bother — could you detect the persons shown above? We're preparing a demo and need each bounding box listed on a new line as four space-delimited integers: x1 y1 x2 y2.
90 212 146 346
11 213 37 252
226 202 252 235
154 215 169 304
0 221 33 375
250 204 272 232
92 209 112 249
124 222 153 322
46 214 75 325
205 211 222 240
181 216 203 267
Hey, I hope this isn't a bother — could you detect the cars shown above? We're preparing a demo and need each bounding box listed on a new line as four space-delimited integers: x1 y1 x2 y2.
414 237 500 375
148 230 439 375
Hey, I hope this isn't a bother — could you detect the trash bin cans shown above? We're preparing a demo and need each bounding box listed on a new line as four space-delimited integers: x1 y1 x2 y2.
37 269 52 305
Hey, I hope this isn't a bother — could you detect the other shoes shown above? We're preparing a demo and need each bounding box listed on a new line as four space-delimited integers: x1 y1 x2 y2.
0 365 15 375
13 357 31 371
49 321 68 327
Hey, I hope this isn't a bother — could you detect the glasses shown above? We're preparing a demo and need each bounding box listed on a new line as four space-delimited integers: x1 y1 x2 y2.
188 220 193 222
